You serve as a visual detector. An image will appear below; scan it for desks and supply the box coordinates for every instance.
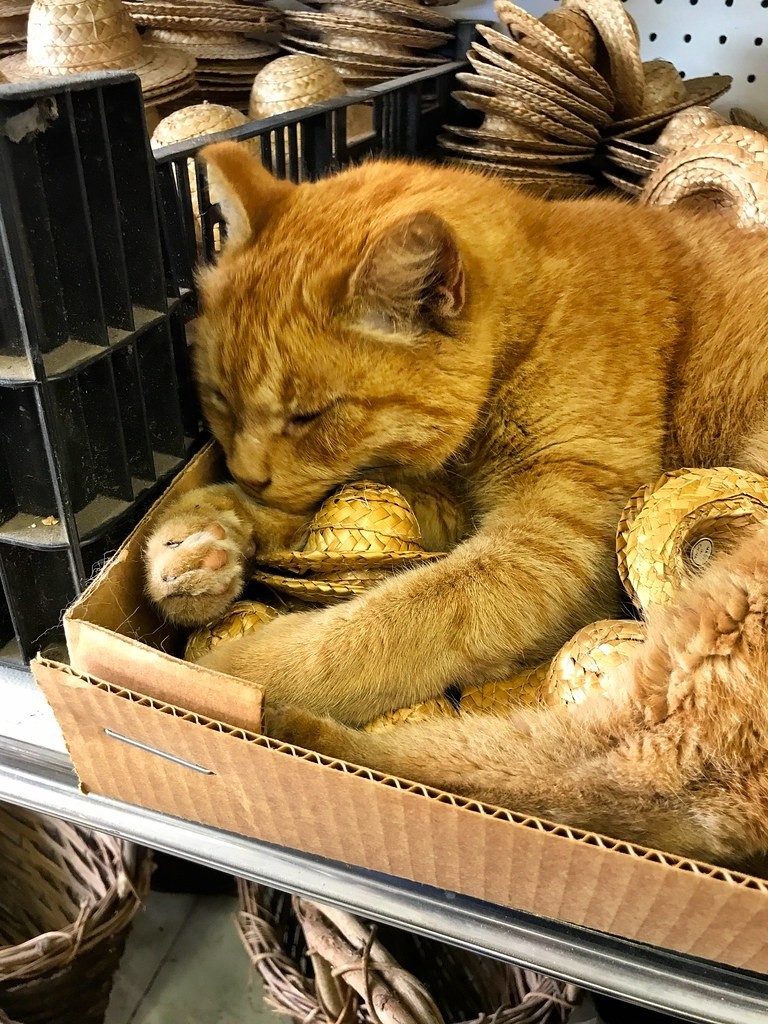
[0,654,768,1024]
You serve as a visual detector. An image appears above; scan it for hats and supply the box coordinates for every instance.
[248,471,449,606]
[457,618,650,719]
[0,0,455,252]
[356,692,461,734]
[434,0,768,230]
[616,465,768,618]
[184,599,286,666]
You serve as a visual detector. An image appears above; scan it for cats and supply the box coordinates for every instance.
[145,142,768,861]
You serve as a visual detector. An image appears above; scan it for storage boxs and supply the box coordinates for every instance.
[28,438,768,975]
[0,61,472,669]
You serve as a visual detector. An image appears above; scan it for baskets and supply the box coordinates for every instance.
[0,800,154,1024]
[229,873,579,1024]
